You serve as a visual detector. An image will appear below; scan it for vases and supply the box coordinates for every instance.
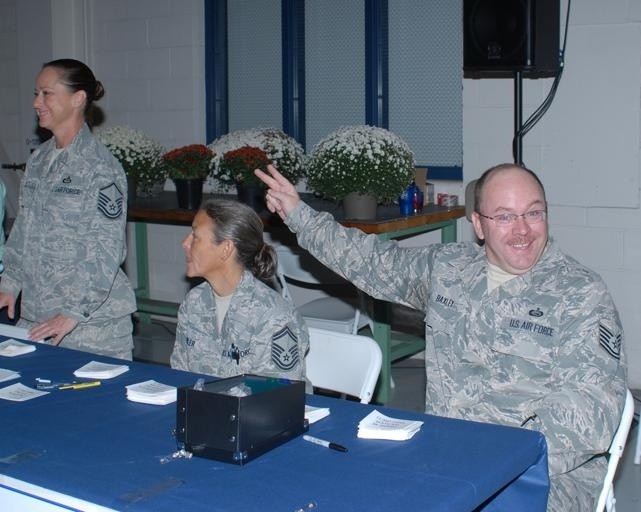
[173,179,205,210]
[126,177,137,205]
[341,190,378,221]
[233,184,265,214]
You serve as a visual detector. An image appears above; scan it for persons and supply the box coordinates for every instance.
[254,164,629,511]
[168,199,313,395]
[1,58,139,361]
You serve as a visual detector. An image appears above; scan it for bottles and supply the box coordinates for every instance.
[399,181,424,219]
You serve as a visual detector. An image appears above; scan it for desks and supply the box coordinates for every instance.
[119,190,463,406]
[0,332,551,512]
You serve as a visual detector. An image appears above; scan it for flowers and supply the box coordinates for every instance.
[160,143,214,179]
[208,130,306,187]
[303,124,416,202]
[91,123,167,184]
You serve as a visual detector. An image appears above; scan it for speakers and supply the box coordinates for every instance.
[463,0,560,79]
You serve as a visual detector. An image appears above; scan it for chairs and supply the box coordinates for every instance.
[591,388,633,512]
[302,326,384,407]
[270,243,395,403]
[0,323,47,344]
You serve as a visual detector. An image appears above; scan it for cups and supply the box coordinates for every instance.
[437,193,458,208]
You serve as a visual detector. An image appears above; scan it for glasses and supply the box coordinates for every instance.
[478,206,550,228]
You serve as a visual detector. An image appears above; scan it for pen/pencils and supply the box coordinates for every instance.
[59,380,101,390]
[303,434,348,452]
[34,383,72,390]
